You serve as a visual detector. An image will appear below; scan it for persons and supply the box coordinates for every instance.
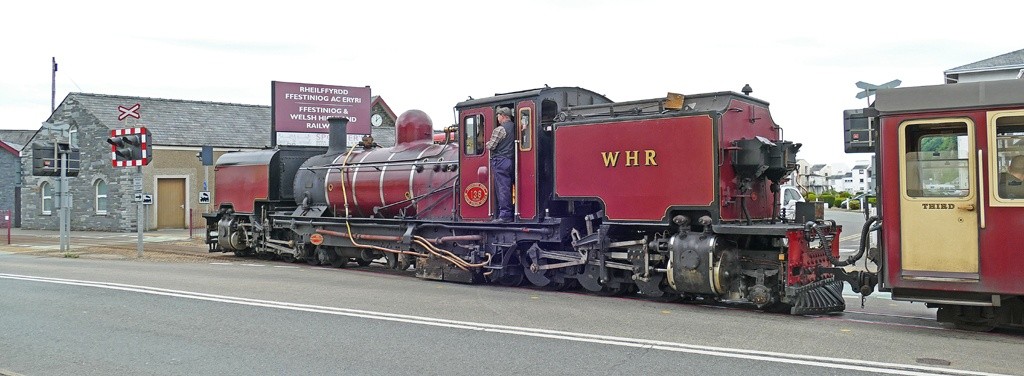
[486,107,515,222]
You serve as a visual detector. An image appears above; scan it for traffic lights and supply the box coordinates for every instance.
[107,127,153,168]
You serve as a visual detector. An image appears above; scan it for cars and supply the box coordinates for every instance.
[841,192,874,210]
[780,184,806,222]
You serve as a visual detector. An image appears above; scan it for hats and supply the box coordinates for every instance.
[496,107,512,116]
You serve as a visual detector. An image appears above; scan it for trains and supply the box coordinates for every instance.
[199,77,1024,340]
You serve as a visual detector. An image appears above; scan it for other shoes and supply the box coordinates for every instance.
[492,218,511,223]
